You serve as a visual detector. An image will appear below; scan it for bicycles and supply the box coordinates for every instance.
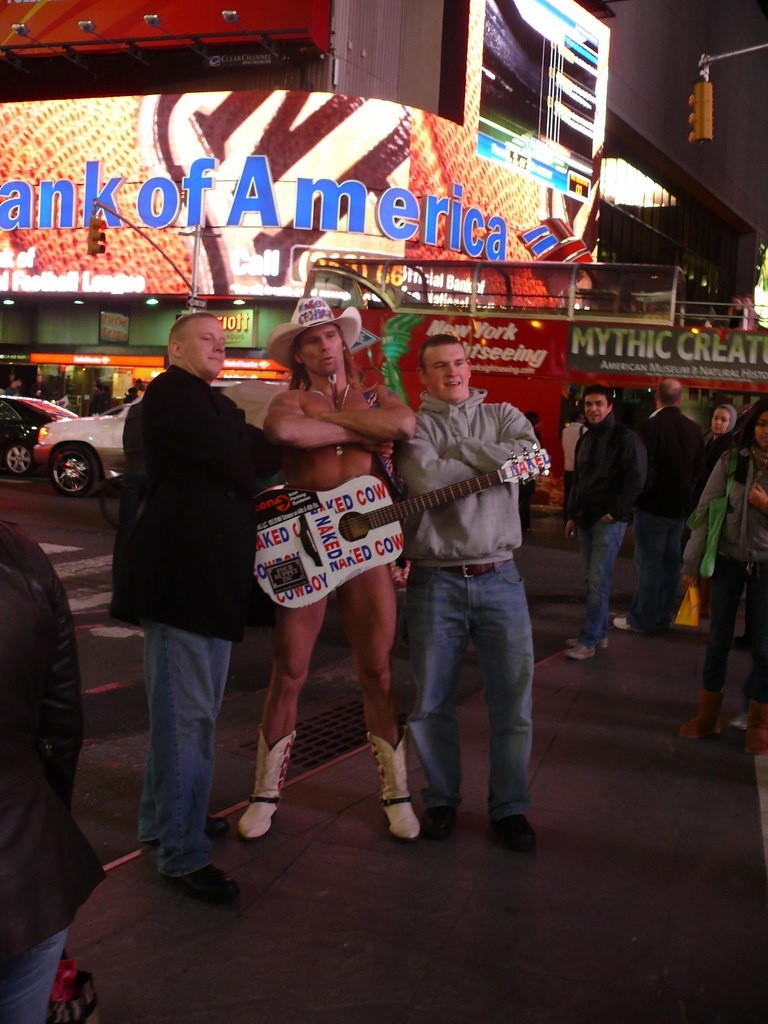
[99,472,127,531]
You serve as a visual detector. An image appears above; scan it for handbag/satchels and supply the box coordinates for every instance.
[673,579,702,628]
[698,448,737,577]
[44,948,98,1024]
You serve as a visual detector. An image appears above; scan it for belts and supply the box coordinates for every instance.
[436,559,507,580]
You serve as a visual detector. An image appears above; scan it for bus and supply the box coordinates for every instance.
[294,250,768,517]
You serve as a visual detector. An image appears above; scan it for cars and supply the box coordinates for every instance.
[0,395,84,479]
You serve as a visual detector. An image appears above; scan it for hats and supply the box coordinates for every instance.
[267,296,362,368]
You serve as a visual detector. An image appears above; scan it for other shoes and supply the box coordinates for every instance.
[566,639,608,660]
[613,618,645,632]
[202,814,231,839]
[491,814,537,850]
[422,805,458,842]
[152,862,241,904]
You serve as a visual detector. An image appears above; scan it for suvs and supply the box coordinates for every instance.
[30,382,292,496]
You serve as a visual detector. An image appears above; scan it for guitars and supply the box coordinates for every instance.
[253,443,550,612]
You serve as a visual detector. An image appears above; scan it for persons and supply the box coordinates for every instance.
[680,399,768,754]
[739,293,757,329]
[388,332,541,854]
[30,374,51,401]
[88,382,111,416]
[705,403,737,480]
[4,377,22,397]
[124,378,146,404]
[613,378,707,635]
[106,308,284,906]
[238,294,423,844]
[519,411,542,532]
[562,405,589,526]
[565,384,649,660]
[727,295,743,330]
[0,522,109,1024]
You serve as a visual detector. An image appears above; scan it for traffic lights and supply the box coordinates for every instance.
[689,80,715,145]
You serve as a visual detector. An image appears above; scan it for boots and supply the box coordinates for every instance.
[238,725,296,841]
[744,697,768,755]
[678,688,724,738]
[367,725,421,842]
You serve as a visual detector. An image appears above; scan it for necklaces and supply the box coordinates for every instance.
[308,384,351,455]
